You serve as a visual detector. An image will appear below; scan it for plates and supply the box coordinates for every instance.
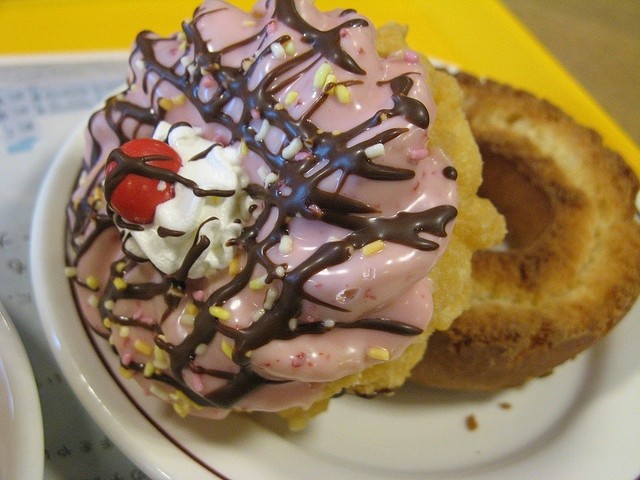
[0,300,46,480]
[30,85,640,480]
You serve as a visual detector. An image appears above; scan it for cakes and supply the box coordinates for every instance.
[65,1,507,431]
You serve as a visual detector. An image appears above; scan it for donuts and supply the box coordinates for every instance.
[407,66,640,391]
